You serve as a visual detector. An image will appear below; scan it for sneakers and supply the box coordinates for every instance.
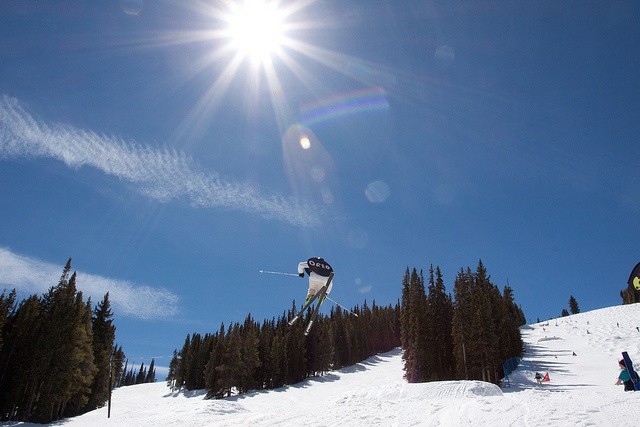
[316,293,325,305]
[305,293,314,305]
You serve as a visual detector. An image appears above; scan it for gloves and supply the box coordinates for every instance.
[299,273,304,278]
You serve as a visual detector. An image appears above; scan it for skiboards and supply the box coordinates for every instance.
[287,272,334,336]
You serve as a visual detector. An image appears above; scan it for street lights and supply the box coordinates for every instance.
[108,350,113,418]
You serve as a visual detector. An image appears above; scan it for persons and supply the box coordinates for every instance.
[632,274,640,303]
[298,257,333,304]
[614,359,640,391]
[534,372,542,385]
